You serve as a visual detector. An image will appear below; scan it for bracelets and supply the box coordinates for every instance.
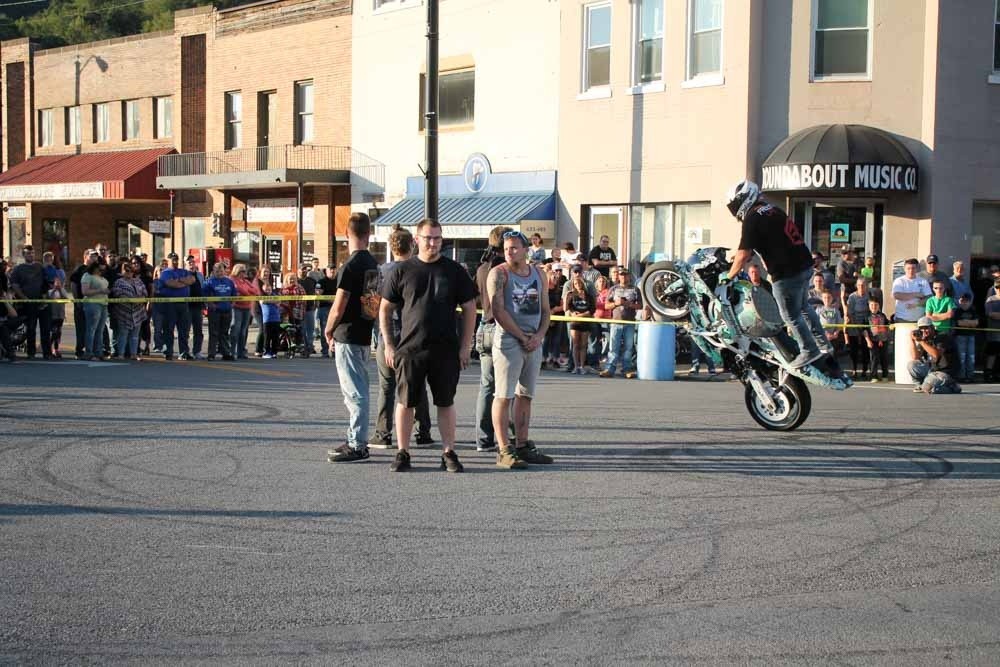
[614,302,616,307]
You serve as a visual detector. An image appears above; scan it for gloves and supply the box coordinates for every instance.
[718,271,733,285]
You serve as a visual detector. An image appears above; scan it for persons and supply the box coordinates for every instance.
[327,212,553,473]
[730,244,1000,383]
[907,317,962,393]
[718,179,833,369]
[460,233,720,379]
[0,240,378,365]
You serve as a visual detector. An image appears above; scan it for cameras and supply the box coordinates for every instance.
[913,330,922,337]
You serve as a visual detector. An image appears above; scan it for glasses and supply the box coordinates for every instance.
[555,269,562,272]
[503,231,527,244]
[417,234,442,241]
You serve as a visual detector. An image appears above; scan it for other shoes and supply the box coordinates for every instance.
[688,358,741,382]
[27,349,335,360]
[541,361,635,378]
[852,371,888,383]
[913,386,925,392]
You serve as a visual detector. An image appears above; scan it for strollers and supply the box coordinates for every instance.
[276,326,310,358]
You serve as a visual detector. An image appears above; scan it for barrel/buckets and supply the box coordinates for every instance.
[635,321,676,380]
[893,323,927,385]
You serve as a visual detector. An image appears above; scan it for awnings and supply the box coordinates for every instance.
[373,193,555,243]
[0,147,175,200]
[761,123,918,198]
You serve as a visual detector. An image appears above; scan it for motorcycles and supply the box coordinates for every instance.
[640,246,811,431]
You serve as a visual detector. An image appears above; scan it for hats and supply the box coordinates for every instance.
[918,317,933,326]
[573,265,582,273]
[552,263,562,270]
[298,263,336,269]
[84,248,98,256]
[927,255,938,264]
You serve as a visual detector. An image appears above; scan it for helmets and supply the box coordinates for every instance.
[727,181,768,221]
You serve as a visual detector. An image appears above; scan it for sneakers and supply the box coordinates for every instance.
[790,349,823,369]
[367,430,392,448]
[819,344,834,353]
[414,434,438,447]
[328,439,370,463]
[477,442,499,452]
[390,449,412,471]
[496,444,528,469]
[440,450,464,473]
[515,440,554,464]
